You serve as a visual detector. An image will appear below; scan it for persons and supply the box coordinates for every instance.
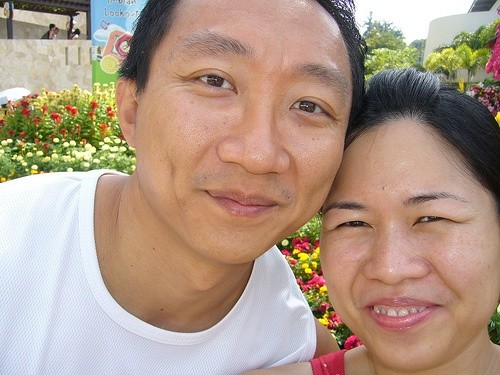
[0,0,370,374]
[41,23,80,40]
[236,67,500,375]
